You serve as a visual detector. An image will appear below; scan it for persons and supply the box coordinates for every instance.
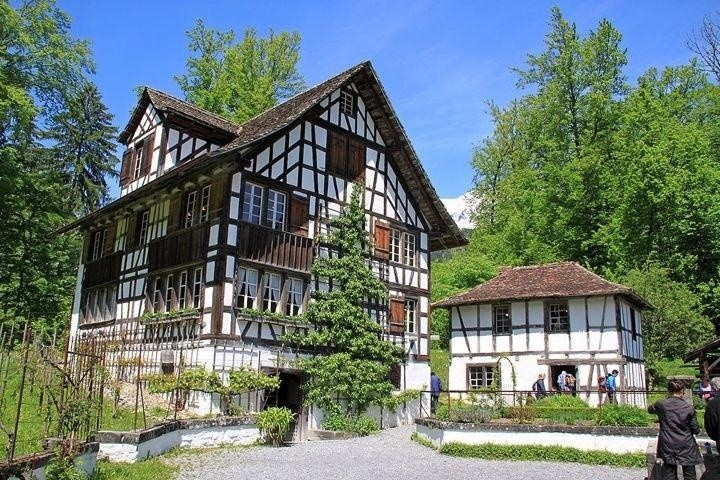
[704,376,720,454]
[431,371,443,414]
[648,379,701,480]
[532,373,547,399]
[557,370,575,395]
[606,370,619,405]
